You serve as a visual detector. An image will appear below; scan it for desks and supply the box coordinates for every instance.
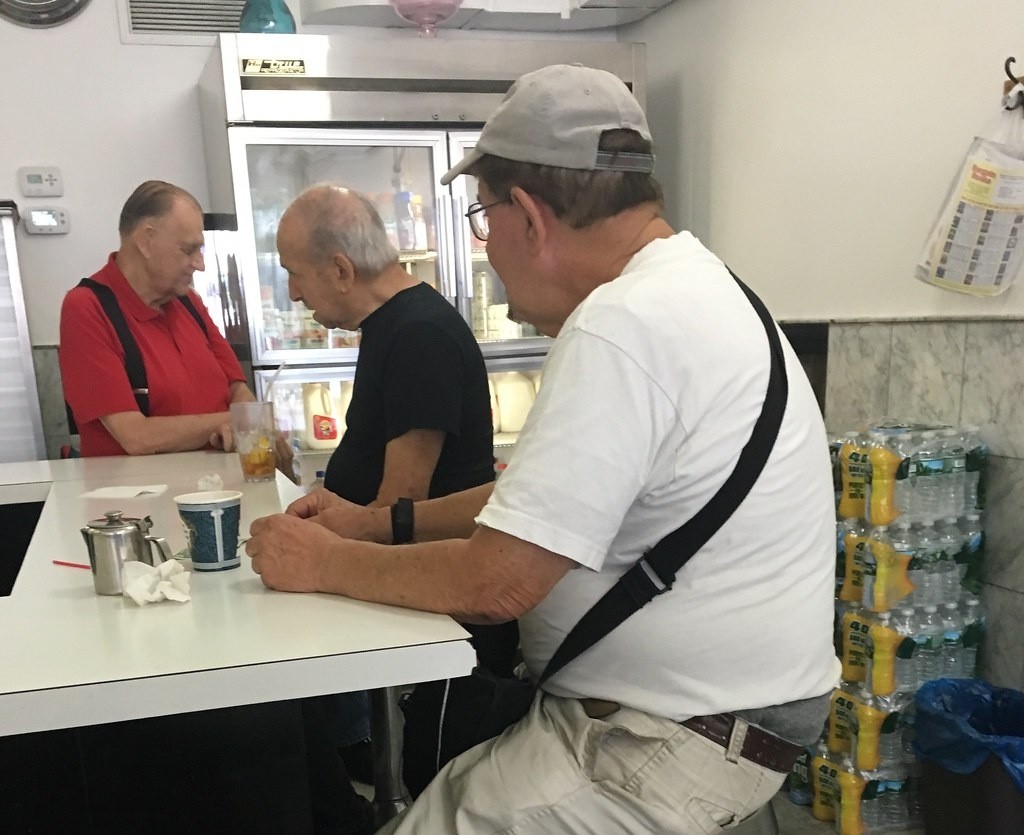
[0,449,479,831]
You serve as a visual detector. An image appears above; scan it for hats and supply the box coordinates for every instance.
[440,62,657,185]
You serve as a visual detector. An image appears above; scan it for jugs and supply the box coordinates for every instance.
[80,511,173,595]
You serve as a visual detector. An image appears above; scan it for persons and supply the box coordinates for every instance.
[245,63,842,835]
[59,180,294,466]
[276,184,496,508]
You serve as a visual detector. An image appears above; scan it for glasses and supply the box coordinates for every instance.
[465,198,509,241]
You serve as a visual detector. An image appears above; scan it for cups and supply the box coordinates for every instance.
[230,402,276,482]
[173,491,243,572]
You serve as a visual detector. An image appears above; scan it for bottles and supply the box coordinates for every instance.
[239,0,297,34]
[487,370,542,433]
[783,407,987,834]
[250,183,440,350]
[308,471,325,491]
[301,380,356,450]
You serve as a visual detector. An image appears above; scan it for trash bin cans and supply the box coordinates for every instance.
[916,679,1024,835]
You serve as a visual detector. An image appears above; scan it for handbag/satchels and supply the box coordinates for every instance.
[406,666,536,802]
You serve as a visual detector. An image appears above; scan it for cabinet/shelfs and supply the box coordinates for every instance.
[229,118,560,476]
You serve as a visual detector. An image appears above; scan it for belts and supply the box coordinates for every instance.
[580,698,803,772]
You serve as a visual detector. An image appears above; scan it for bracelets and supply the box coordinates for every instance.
[390,498,414,543]
